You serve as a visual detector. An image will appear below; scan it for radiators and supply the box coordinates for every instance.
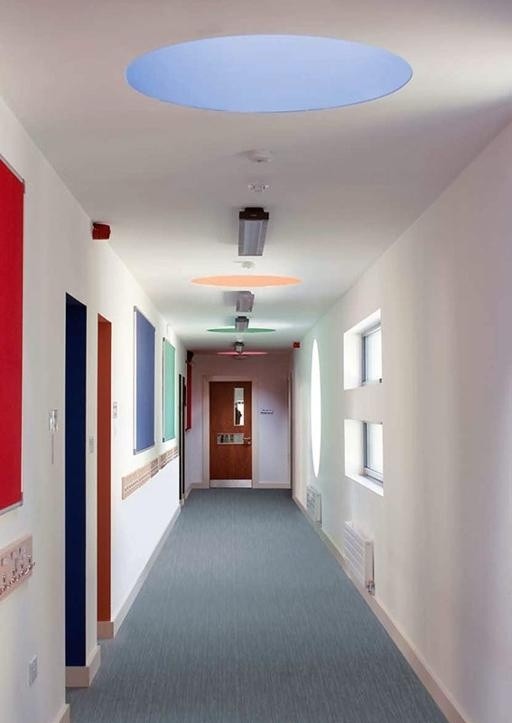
[304,485,322,525]
[340,519,375,598]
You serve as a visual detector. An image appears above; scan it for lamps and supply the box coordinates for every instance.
[233,316,250,333]
[235,202,270,258]
[233,341,245,354]
[233,291,255,312]
